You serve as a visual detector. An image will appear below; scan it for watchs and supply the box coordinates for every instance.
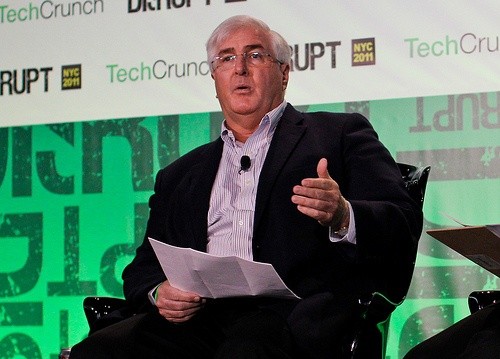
[331,200,351,237]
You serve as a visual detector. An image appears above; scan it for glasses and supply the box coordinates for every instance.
[209,52,284,69]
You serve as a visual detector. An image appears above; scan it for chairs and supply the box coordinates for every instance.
[56,163,431,359]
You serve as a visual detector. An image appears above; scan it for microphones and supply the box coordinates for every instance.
[238,155,251,175]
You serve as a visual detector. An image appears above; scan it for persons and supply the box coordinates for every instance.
[403,297,500,359]
[66,13,424,359]
